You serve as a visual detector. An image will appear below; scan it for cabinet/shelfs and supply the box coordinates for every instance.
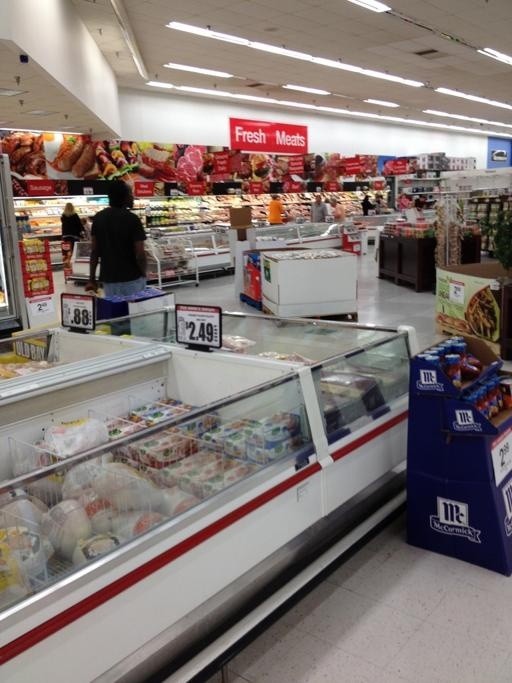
[0,304,423,683]
[405,334,512,577]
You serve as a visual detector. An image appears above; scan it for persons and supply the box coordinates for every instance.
[311,191,332,222]
[373,193,387,215]
[361,195,376,216]
[268,194,292,225]
[86,179,146,296]
[330,198,345,222]
[413,196,426,209]
[394,191,411,213]
[60,202,97,261]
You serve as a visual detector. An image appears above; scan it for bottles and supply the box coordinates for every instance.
[413,334,512,422]
[144,199,184,226]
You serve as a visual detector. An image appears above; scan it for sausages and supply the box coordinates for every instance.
[72,145,94,177]
[58,140,82,170]
